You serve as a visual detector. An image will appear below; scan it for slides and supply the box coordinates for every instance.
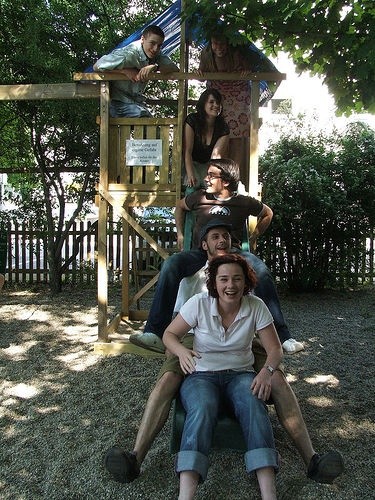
[168,187,250,453]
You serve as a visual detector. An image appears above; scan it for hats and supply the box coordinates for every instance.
[199,220,233,240]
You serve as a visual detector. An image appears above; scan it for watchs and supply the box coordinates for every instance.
[262,365,275,376]
[154,62,161,72]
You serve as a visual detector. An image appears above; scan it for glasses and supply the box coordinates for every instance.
[206,173,225,179]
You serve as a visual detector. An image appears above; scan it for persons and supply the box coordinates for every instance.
[197,28,259,181]
[92,24,180,182]
[102,218,345,485]
[162,252,285,500]
[181,88,232,193]
[128,158,306,355]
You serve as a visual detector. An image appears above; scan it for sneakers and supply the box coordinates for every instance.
[129,332,166,354]
[282,338,305,355]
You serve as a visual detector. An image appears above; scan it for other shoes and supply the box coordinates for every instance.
[104,448,140,483]
[309,450,344,483]
